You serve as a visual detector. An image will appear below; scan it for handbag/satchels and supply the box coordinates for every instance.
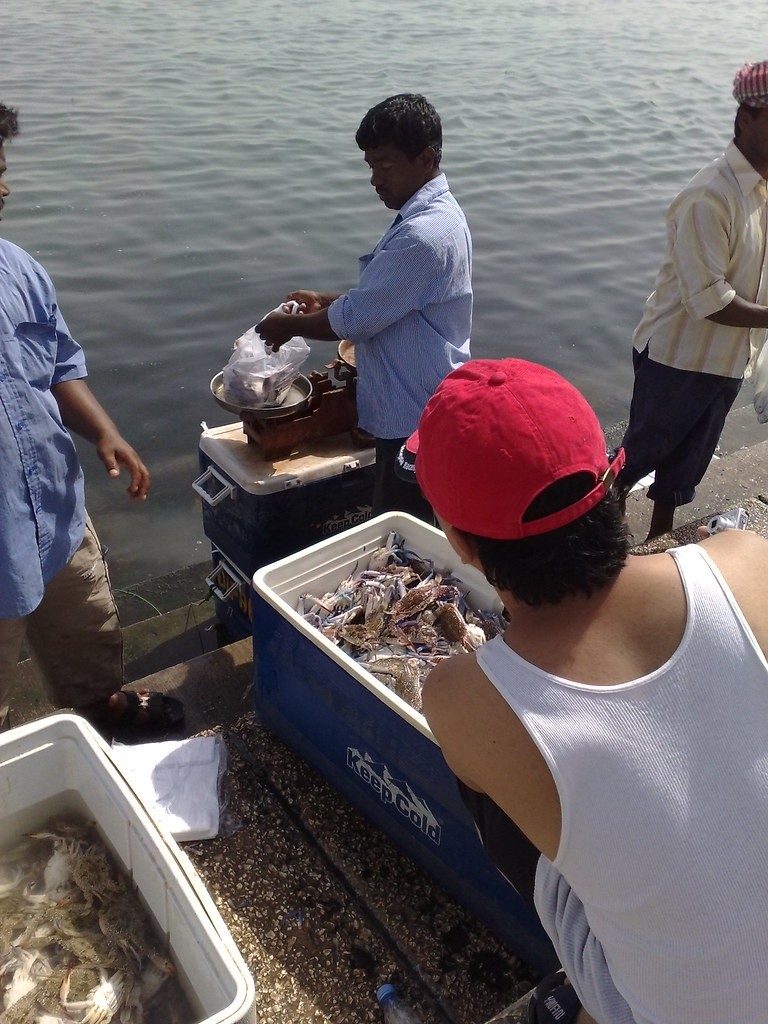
[224,299,308,408]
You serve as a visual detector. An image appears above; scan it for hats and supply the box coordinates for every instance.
[393,359,626,540]
[732,59,768,107]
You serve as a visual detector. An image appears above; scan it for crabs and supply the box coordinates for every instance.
[0,817,174,1024]
[296,532,509,713]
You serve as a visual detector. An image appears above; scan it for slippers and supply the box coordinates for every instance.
[528,972,584,1024]
[96,691,185,730]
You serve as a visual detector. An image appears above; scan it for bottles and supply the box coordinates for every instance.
[376,983,424,1024]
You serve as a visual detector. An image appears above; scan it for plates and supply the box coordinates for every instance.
[210,368,313,417]
[338,338,356,371]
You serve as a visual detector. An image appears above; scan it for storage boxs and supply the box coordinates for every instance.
[191,420,376,579]
[0,713,256,1024]
[251,512,561,976]
[206,541,253,640]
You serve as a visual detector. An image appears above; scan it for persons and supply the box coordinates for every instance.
[609,59,768,543]
[254,94,475,530]
[0,101,187,737]
[405,356,768,1024]
